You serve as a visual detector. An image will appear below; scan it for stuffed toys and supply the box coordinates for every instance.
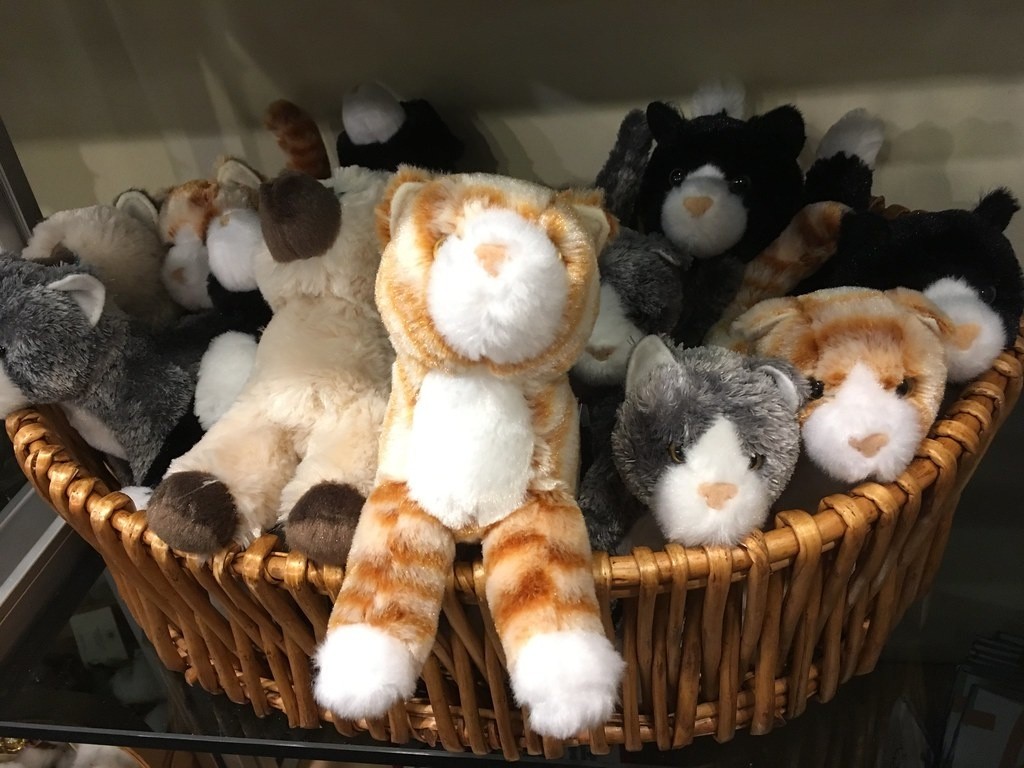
[0,76,1024,738]
[311,163,632,738]
[145,163,389,566]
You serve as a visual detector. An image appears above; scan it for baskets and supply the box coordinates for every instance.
[6,315,1024,761]
